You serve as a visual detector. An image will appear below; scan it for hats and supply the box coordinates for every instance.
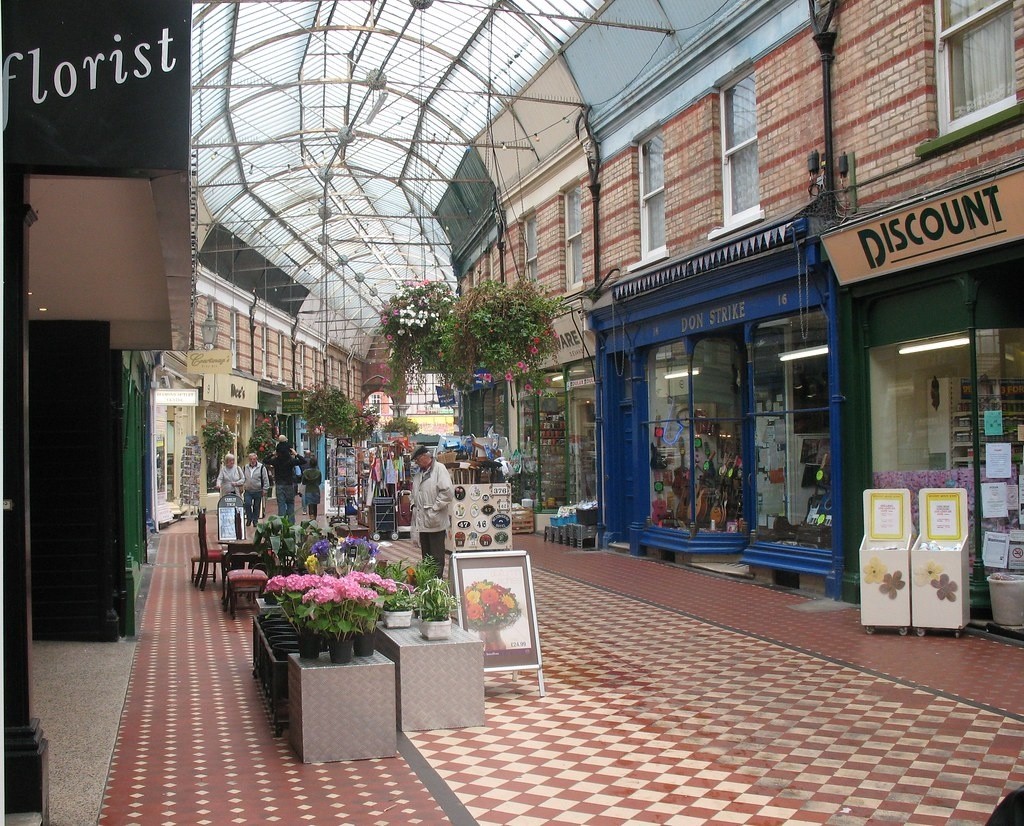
[410,446,428,461]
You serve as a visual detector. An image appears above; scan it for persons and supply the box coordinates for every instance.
[410,446,455,578]
[217,453,269,527]
[263,435,322,525]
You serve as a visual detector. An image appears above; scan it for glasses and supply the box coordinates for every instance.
[414,455,422,462]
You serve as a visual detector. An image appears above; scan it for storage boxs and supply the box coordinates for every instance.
[549,507,598,526]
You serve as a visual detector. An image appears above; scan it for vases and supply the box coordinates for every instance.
[329,639,353,664]
[298,639,320,658]
[255,613,303,661]
[312,633,327,652]
[352,632,374,657]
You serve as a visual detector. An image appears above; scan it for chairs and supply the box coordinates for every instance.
[195,507,230,591]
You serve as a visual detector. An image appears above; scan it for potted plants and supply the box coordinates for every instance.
[380,581,416,629]
[418,588,453,640]
[420,578,453,616]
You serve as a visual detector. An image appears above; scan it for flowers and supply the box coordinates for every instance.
[265,572,398,640]
[303,535,381,577]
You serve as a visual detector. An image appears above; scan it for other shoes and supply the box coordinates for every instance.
[303,512,307,515]
[253,523,257,527]
[246,520,252,526]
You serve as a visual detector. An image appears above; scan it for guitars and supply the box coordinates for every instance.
[803,454,832,526]
[651,436,732,529]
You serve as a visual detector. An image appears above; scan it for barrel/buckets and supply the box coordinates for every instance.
[987,575,1024,625]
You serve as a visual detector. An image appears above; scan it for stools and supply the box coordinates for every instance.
[190,556,216,584]
[222,566,268,620]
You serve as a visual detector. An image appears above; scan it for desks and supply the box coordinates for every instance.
[217,540,266,598]
